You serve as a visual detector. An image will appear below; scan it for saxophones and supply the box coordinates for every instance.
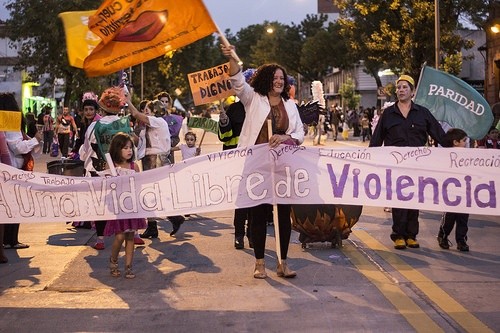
[24,125,44,157]
[110,71,131,109]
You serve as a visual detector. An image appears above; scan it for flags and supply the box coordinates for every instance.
[60,10,102,68]
[82,0,220,77]
[414,65,494,140]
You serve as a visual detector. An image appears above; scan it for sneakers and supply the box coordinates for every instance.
[406,238,419,247]
[457,241,469,251]
[394,239,406,248]
[439,239,449,249]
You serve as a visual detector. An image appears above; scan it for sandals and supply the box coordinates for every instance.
[277,259,297,276]
[110,256,120,276]
[254,259,266,278]
[3,240,29,248]
[125,265,135,278]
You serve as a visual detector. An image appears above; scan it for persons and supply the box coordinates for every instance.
[360,74,448,250]
[25,106,85,157]
[471,102,500,149]
[300,100,386,147]
[0,131,12,263]
[219,68,255,249]
[103,131,147,279]
[437,128,472,252]
[156,93,183,164]
[126,93,185,239]
[217,43,304,279]
[133,100,150,136]
[171,132,201,217]
[91,87,145,250]
[67,93,102,229]
[267,75,308,226]
[55,106,79,158]
[0,92,44,248]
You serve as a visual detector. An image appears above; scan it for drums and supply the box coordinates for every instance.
[45,158,85,178]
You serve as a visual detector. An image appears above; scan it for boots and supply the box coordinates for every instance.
[167,215,185,236]
[139,221,158,238]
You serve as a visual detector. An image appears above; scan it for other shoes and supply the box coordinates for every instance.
[0,255,8,263]
[95,239,104,249]
[250,240,254,248]
[235,239,244,248]
[134,235,145,244]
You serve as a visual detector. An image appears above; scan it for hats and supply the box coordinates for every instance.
[397,75,414,86]
